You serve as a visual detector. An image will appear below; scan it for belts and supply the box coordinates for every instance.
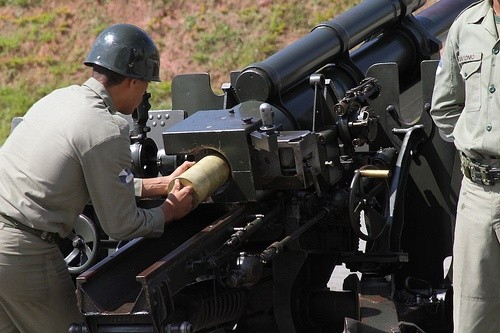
[459,150,500,186]
[0,212,59,244]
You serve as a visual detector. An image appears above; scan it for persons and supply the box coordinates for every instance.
[428,0,499,333]
[0,23,196,333]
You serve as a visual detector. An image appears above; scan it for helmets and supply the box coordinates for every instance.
[83,23,162,82]
